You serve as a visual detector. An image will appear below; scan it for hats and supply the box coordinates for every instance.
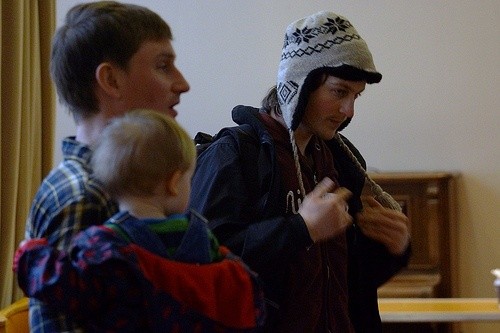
[276,10,403,211]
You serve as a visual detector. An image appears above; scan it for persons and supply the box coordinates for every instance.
[12,0,268,333]
[190,11,413,333]
